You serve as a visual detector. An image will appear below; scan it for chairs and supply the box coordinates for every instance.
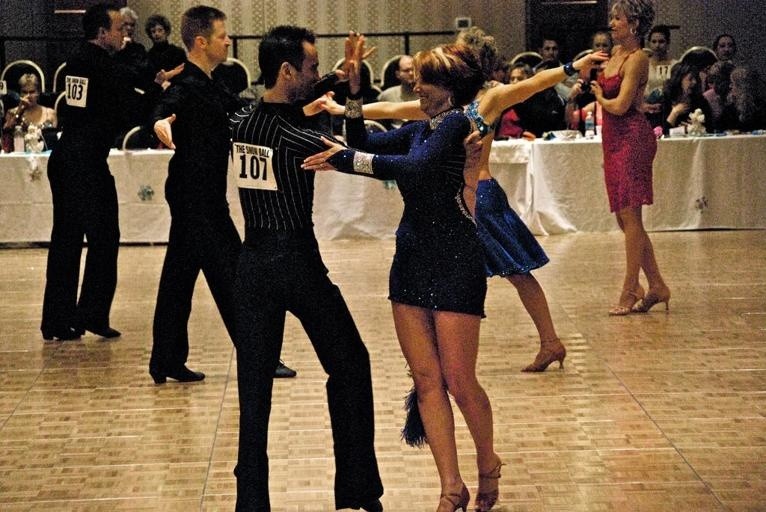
[679,46,718,70]
[1,60,46,95]
[0,134,766,246]
[379,54,402,92]
[511,52,543,67]
[55,91,66,125]
[573,49,592,61]
[364,119,388,133]
[122,125,158,149]
[53,63,67,92]
[210,57,251,94]
[331,58,381,106]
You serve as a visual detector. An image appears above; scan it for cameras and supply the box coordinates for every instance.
[581,80,592,92]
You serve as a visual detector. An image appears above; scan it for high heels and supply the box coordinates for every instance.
[440,483,470,512]
[336,484,383,512]
[520,337,565,372]
[631,286,671,312]
[474,456,502,512]
[42,326,82,341]
[608,285,645,316]
[149,362,205,384]
[275,362,297,378]
[75,318,121,337]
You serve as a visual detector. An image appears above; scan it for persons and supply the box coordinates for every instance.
[119,5,144,59]
[491,23,764,143]
[3,72,57,132]
[37,2,165,343]
[297,43,506,512]
[144,5,296,386]
[375,54,420,102]
[318,24,611,373]
[226,23,388,511]
[589,1,674,318]
[142,13,184,70]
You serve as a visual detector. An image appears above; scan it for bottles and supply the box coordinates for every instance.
[585,112,595,141]
[14,126,24,152]
